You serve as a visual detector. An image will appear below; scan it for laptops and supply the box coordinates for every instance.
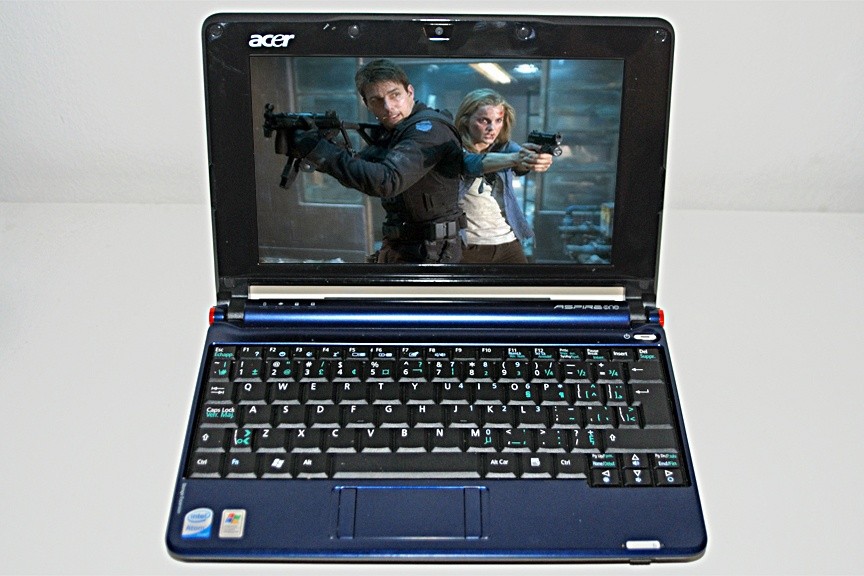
[165,11,708,567]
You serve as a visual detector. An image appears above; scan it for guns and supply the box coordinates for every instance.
[263,102,385,191]
[526,128,565,160]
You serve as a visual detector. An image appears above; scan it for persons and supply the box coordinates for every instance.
[292,58,465,263]
[455,87,553,263]
